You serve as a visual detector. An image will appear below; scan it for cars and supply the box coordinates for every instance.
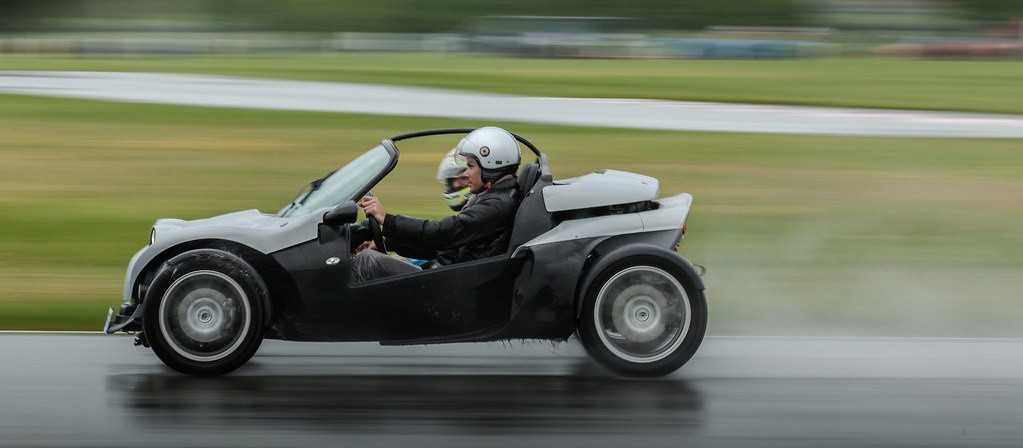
[99,124,711,380]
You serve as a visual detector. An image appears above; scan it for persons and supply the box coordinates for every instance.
[354,146,478,271]
[349,125,525,283]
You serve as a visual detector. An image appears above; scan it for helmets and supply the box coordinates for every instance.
[458,126,522,184]
[438,147,474,211]
